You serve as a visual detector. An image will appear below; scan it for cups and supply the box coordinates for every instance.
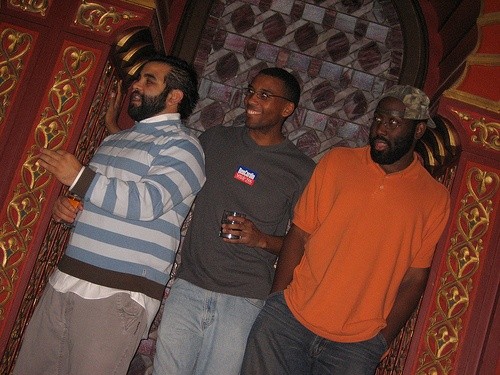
[67,190,81,207]
[221,209,246,239]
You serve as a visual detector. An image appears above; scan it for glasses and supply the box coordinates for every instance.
[369,117,407,129]
[243,88,292,102]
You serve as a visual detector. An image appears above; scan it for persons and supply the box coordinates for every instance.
[150,67,316,374]
[11,56,207,375]
[240,83,450,375]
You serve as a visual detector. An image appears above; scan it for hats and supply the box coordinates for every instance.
[375,83,436,128]
